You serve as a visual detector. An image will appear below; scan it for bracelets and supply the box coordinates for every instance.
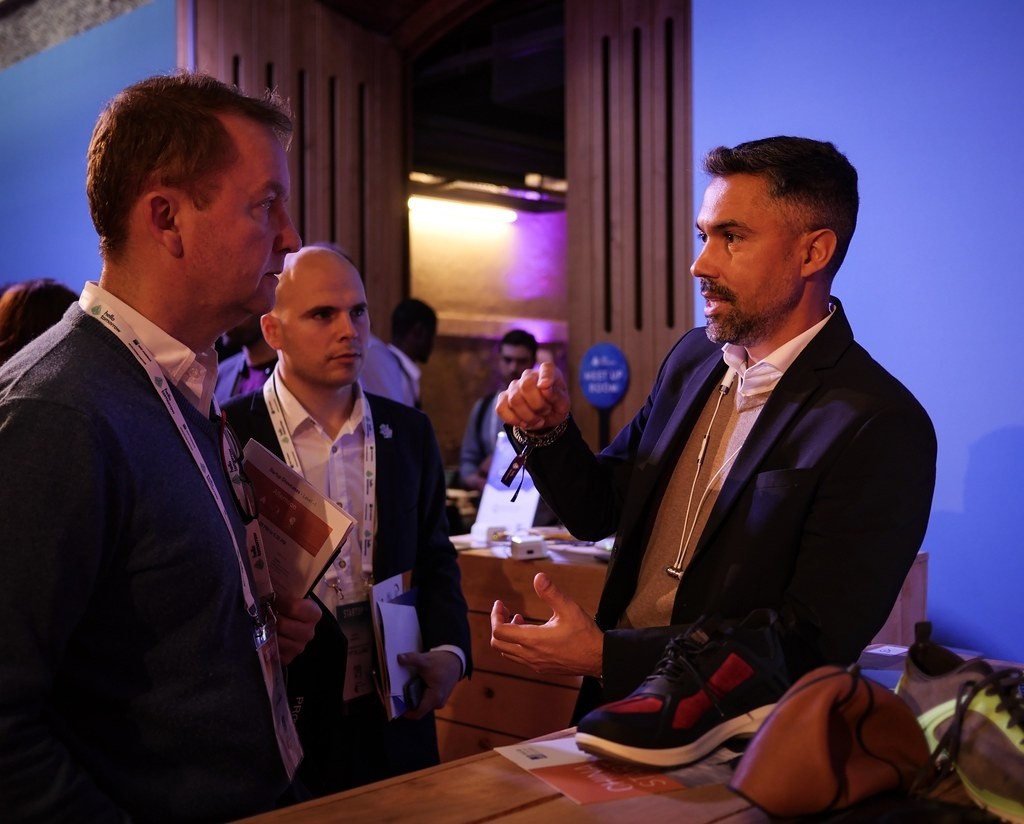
[501,413,573,502]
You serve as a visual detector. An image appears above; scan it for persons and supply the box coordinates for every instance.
[0,279,554,530]
[0,69,304,824]
[221,243,473,798]
[492,136,937,728]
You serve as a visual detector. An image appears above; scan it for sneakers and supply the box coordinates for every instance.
[893,621,1024,824]
[574,613,792,767]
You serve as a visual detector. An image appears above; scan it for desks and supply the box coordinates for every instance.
[226,641,1024,824]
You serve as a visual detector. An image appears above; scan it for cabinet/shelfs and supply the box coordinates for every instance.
[435,526,929,762]
